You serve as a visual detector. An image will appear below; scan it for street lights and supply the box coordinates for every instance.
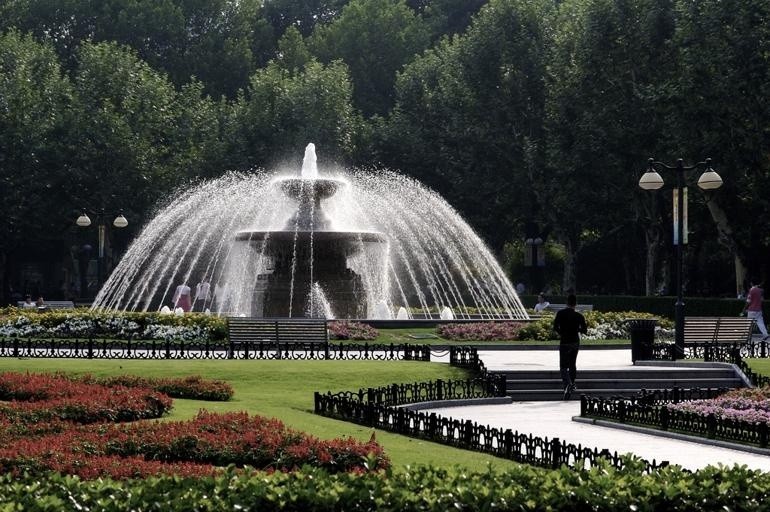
[639,157,727,360]
[525,237,545,297]
[76,206,129,294]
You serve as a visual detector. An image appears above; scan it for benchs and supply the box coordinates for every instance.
[226,316,328,358]
[683,316,755,364]
[546,304,594,317]
[18,300,74,311]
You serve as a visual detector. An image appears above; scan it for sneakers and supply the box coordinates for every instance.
[563,383,577,401]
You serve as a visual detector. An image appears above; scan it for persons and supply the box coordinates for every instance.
[743,279,769,341]
[553,293,587,400]
[533,292,550,312]
[516,280,525,296]
[172,274,229,312]
[22,294,44,309]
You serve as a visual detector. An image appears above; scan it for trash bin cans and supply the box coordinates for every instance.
[626,318,659,365]
